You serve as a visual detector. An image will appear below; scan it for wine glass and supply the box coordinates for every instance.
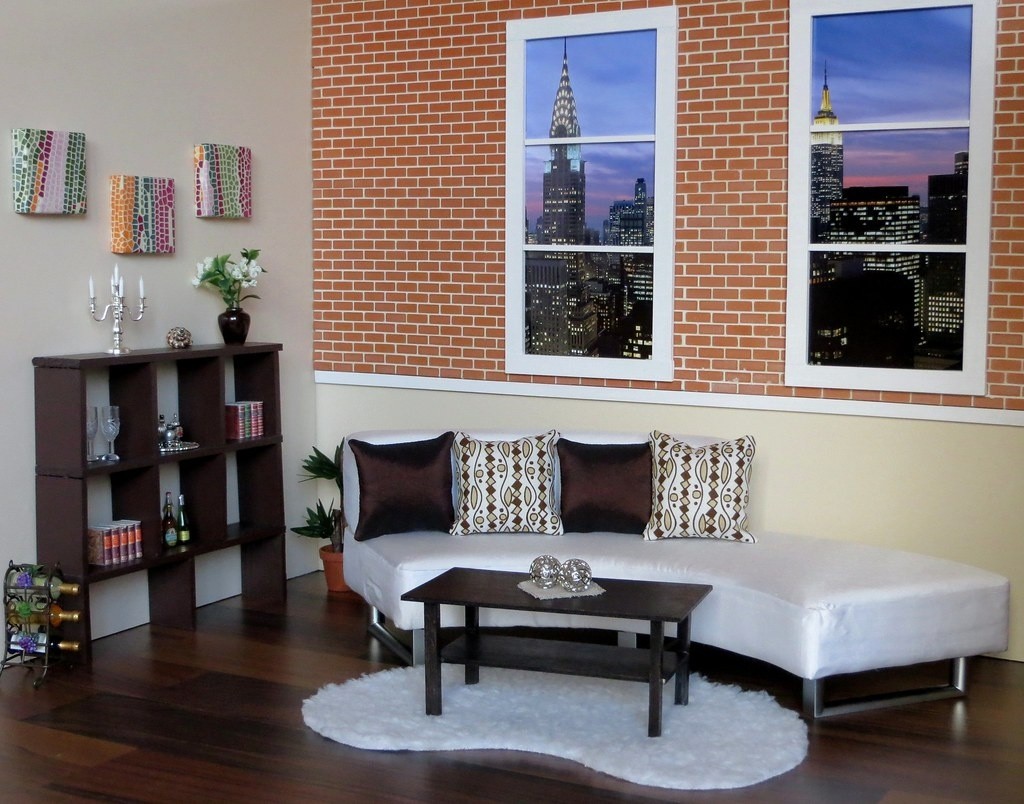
[99,406,122,461]
[84,405,100,461]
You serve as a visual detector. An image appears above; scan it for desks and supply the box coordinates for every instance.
[400,567,713,740]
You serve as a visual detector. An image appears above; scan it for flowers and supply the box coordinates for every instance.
[192,248,268,308]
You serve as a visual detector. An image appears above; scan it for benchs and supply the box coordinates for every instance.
[342,431,1010,721]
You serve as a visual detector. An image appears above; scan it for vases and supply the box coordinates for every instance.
[218,307,251,345]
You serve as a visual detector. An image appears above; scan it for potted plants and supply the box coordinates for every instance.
[290,435,353,592]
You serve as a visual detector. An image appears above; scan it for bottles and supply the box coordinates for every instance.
[158,414,183,442]
[176,493,191,546]
[7,571,80,599]
[7,600,81,626]
[8,631,81,655]
[162,491,179,548]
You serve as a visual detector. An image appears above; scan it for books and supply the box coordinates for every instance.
[88,520,142,566]
[225,401,263,438]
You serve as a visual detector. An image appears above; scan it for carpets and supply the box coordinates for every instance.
[301,660,809,790]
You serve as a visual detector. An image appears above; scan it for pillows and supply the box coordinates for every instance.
[348,431,761,546]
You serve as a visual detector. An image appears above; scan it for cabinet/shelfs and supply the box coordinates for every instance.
[32,340,289,681]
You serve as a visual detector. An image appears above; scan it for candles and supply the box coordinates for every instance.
[89,263,144,297]
